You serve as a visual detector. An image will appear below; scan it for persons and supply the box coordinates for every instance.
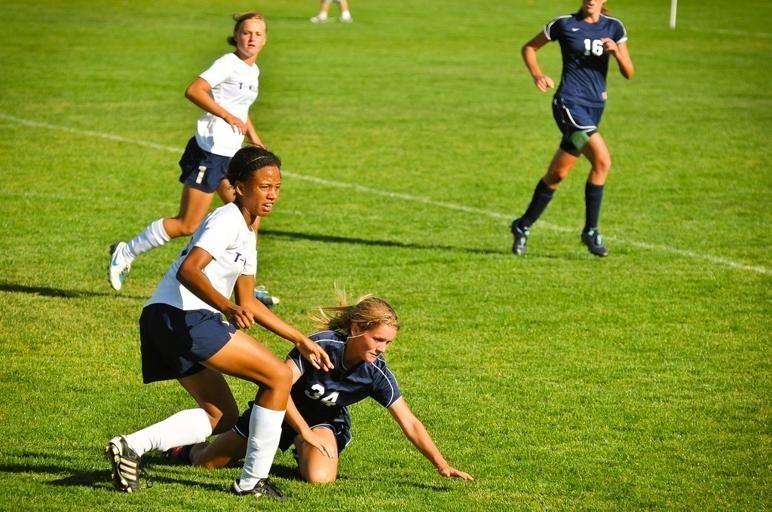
[105,11,281,308]
[101,143,332,502]
[156,282,478,487]
[507,0,635,257]
[307,0,358,24]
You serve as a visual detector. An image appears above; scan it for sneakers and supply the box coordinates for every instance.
[159,442,183,464]
[108,239,134,294]
[105,433,144,497]
[578,226,609,259]
[508,219,530,257]
[228,474,285,499]
[252,284,281,307]
